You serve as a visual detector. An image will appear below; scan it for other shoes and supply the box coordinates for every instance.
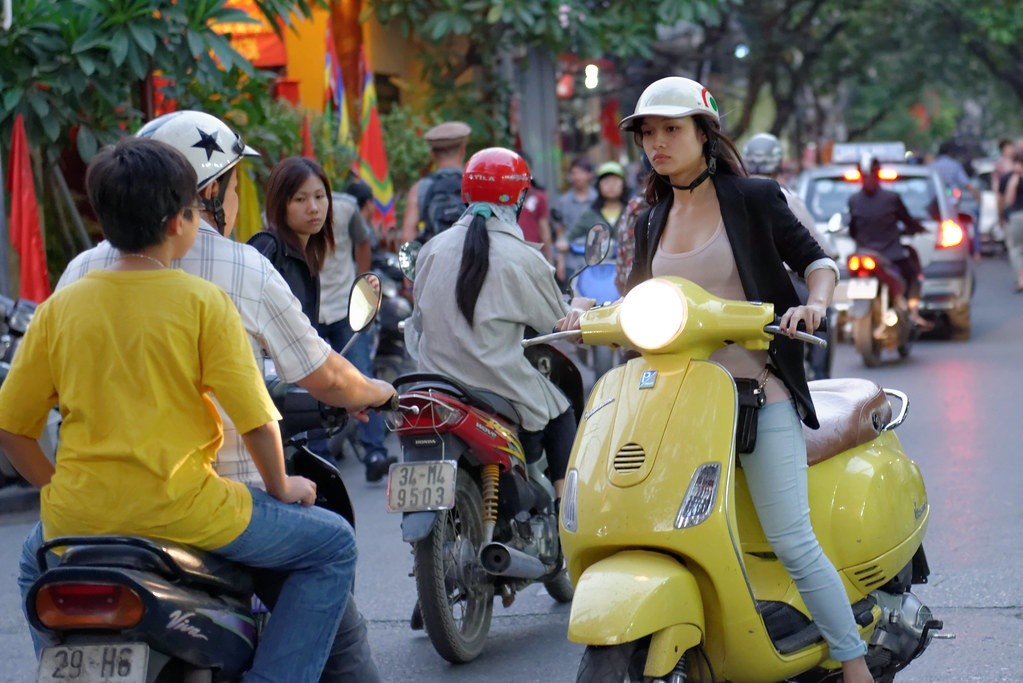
[411,603,424,631]
[908,311,935,329]
[364,452,398,482]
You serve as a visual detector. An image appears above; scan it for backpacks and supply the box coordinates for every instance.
[415,172,468,244]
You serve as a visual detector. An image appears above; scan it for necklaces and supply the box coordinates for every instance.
[120,254,164,267]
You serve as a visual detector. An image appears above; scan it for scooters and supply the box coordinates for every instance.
[0,225,626,683]
[846,227,932,368]
[522,276,956,683]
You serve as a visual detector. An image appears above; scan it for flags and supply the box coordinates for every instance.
[352,47,395,232]
[6,114,51,302]
[323,34,348,147]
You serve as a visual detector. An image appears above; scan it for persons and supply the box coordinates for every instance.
[400,147,595,513]
[743,134,840,381]
[518,152,630,295]
[347,184,379,270]
[558,77,874,682]
[992,139,1023,292]
[245,157,335,335]
[1,139,356,683]
[931,141,982,261]
[400,121,472,302]
[849,154,934,329]
[257,191,385,466]
[54,111,398,682]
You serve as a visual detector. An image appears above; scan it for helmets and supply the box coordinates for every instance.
[616,76,721,132]
[596,161,624,179]
[458,148,530,206]
[132,110,261,192]
[858,154,882,176]
[744,133,781,173]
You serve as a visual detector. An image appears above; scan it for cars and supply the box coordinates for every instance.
[801,140,998,340]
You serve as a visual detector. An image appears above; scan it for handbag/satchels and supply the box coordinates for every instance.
[733,376,766,453]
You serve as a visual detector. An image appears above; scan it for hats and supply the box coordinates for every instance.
[426,122,472,147]
